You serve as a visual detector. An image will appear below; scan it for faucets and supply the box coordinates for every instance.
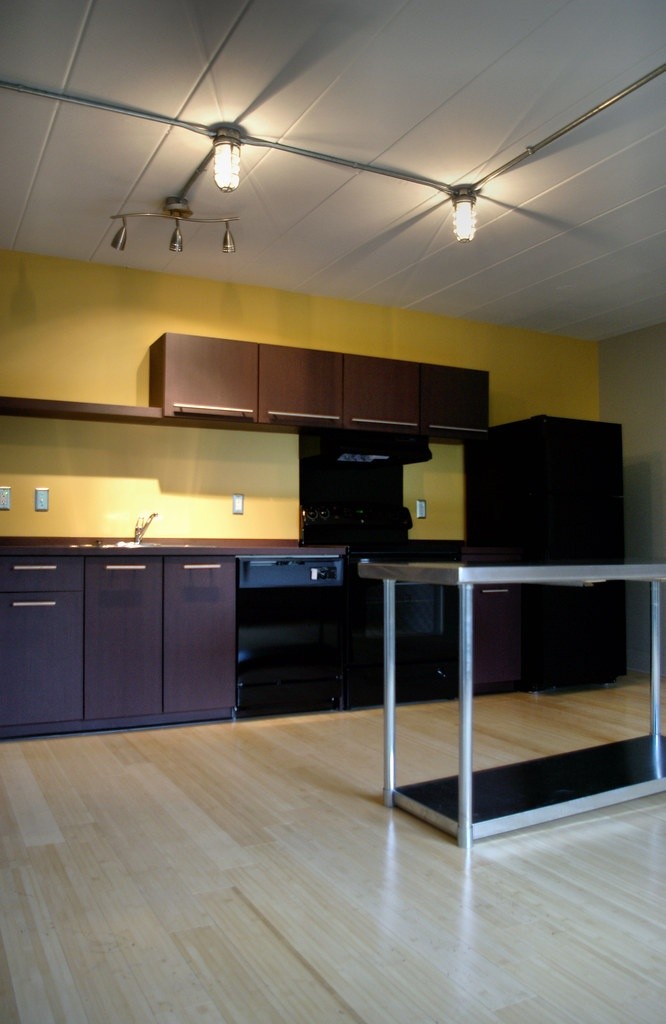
[136,512,158,543]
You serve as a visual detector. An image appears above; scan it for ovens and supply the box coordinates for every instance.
[346,557,460,708]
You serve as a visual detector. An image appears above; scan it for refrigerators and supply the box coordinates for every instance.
[464,415,629,694]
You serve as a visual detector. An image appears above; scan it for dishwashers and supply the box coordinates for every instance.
[233,554,343,718]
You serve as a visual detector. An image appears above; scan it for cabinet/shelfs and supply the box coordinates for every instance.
[463,415,628,693]
[148,332,490,442]
[0,555,236,740]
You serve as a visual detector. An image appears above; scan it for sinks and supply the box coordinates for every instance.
[68,542,212,549]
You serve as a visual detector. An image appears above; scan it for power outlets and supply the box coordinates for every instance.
[0,487,12,511]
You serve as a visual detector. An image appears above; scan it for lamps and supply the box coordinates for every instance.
[212,126,244,194]
[169,218,182,253]
[223,221,236,254]
[451,188,477,243]
[110,217,128,251]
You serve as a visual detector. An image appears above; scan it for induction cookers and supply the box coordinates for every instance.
[302,504,457,558]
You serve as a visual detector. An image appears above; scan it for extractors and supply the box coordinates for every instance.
[299,427,433,466]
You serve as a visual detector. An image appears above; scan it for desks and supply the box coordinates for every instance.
[355,561,665,851]
